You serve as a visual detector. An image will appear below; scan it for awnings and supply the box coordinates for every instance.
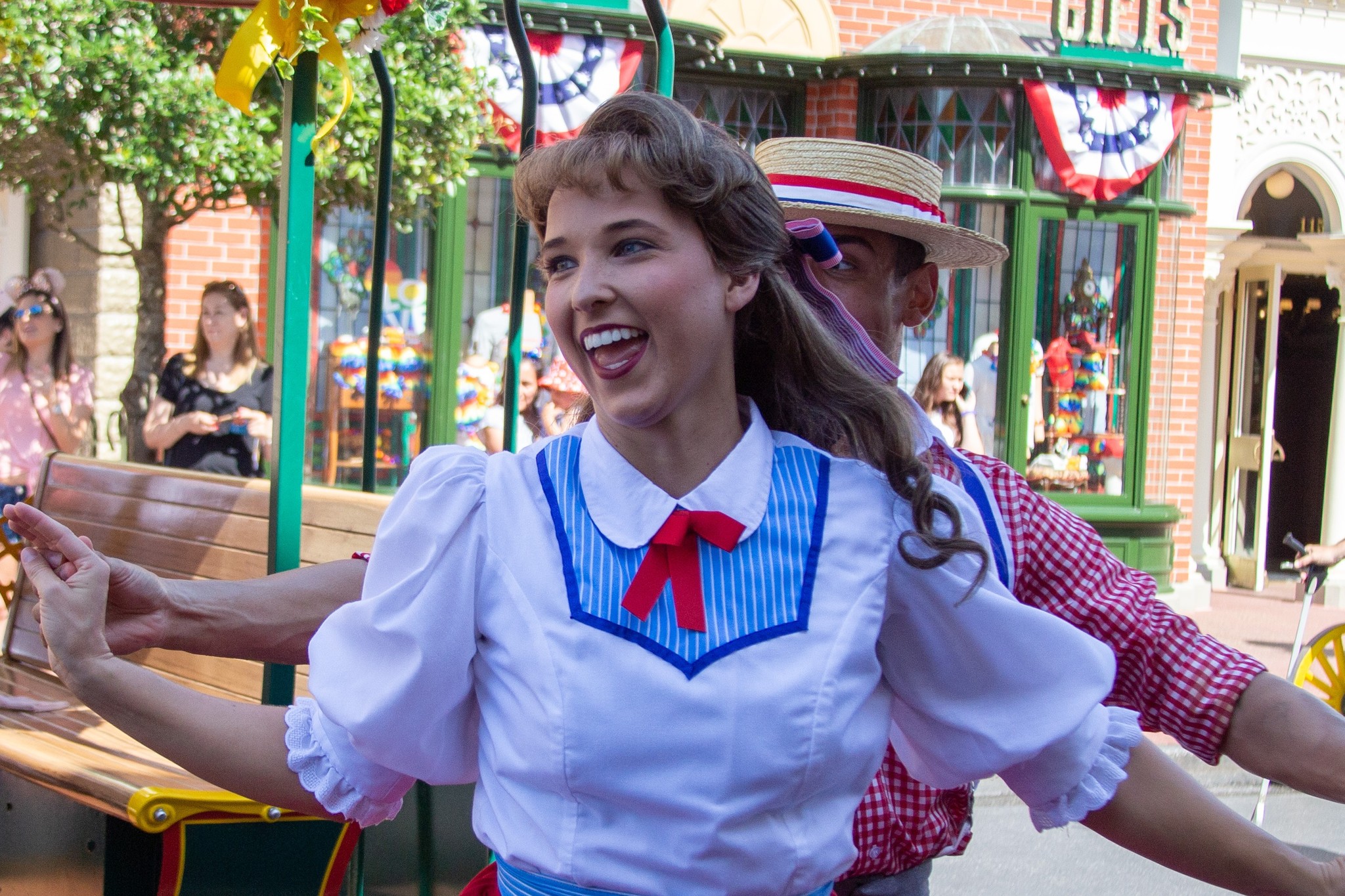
[479,2,1251,105]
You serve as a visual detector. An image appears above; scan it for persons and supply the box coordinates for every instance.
[0,291,94,542]
[0,307,20,354]
[914,351,984,456]
[35,138,1345,896]
[1295,538,1345,584]
[3,93,1345,896]
[142,281,275,478]
[537,354,587,440]
[482,351,545,454]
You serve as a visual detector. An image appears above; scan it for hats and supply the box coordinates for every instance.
[756,136,1010,270]
[538,358,587,396]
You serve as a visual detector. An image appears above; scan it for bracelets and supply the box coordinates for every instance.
[170,417,186,440]
[39,401,63,421]
[959,411,977,418]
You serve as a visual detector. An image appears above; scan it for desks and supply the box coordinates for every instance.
[322,348,427,487]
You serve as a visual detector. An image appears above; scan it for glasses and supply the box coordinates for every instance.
[13,303,56,318]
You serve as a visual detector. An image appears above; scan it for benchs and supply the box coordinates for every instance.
[0,449,399,896]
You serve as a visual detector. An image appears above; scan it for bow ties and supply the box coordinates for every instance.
[621,510,748,634]
